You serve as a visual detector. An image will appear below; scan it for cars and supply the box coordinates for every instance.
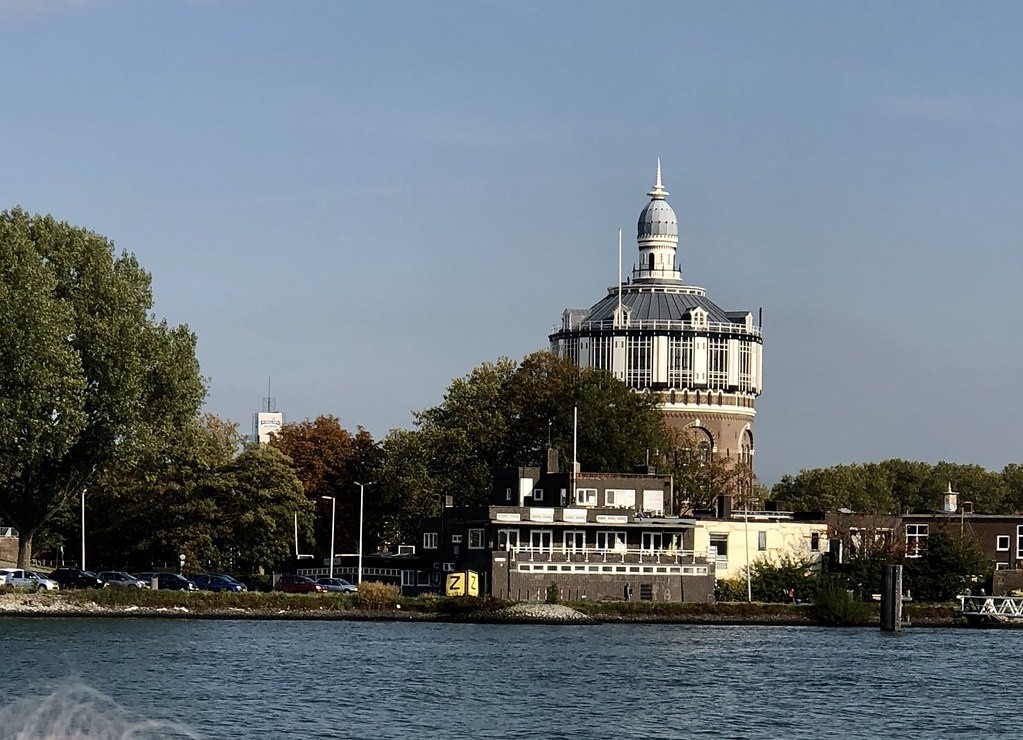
[137,572,199,591]
[194,574,248,592]
[315,578,358,595]
[97,571,150,591]
[276,576,328,594]
[0,568,24,587]
[5,570,60,592]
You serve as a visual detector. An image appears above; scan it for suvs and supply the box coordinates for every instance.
[48,567,110,589]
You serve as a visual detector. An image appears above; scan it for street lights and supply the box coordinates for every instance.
[322,496,336,583]
[353,481,363,584]
[82,489,89,570]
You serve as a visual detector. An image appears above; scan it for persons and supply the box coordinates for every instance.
[978,588,988,613]
[959,588,974,612]
[624,583,632,602]
